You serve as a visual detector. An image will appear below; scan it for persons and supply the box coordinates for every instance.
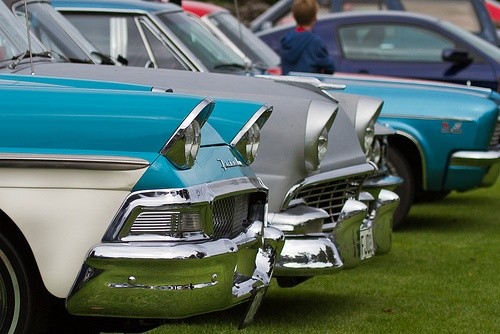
[278,0,336,76]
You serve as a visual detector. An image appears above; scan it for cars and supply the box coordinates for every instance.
[1,0,498,334]
